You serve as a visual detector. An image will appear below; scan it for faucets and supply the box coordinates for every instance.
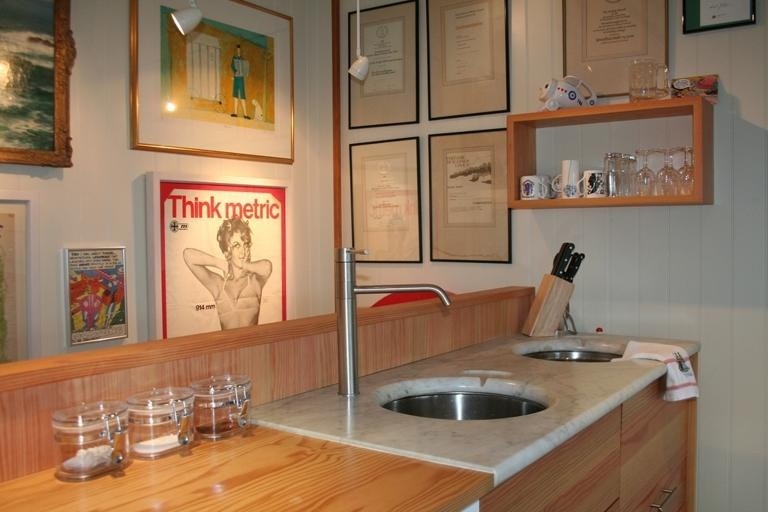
[334,245,452,398]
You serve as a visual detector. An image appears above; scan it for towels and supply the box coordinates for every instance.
[611,340,700,401]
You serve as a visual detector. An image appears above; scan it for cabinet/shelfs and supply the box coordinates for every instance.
[2,284,696,512]
[505,95,713,209]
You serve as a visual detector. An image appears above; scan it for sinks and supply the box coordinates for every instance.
[371,374,556,421]
[509,338,628,364]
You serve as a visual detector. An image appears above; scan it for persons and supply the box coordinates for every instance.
[230,44,251,119]
[184,218,272,330]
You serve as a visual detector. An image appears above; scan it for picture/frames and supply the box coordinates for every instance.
[563,1,669,99]
[64,245,130,346]
[143,171,287,342]
[129,0,296,166]
[348,136,422,265]
[0,198,27,363]
[0,0,77,168]
[427,129,515,265]
[680,0,760,36]
[347,2,419,130]
[426,0,512,120]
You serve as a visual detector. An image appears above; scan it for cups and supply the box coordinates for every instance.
[519,176,547,201]
[552,159,582,199]
[654,145,679,196]
[575,169,607,198]
[604,153,623,197]
[685,148,694,195]
[635,148,655,196]
[628,58,669,101]
[620,154,636,195]
[537,174,556,198]
[680,145,688,192]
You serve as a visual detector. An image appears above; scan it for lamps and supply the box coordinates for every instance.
[172,0,206,36]
[347,0,370,82]
